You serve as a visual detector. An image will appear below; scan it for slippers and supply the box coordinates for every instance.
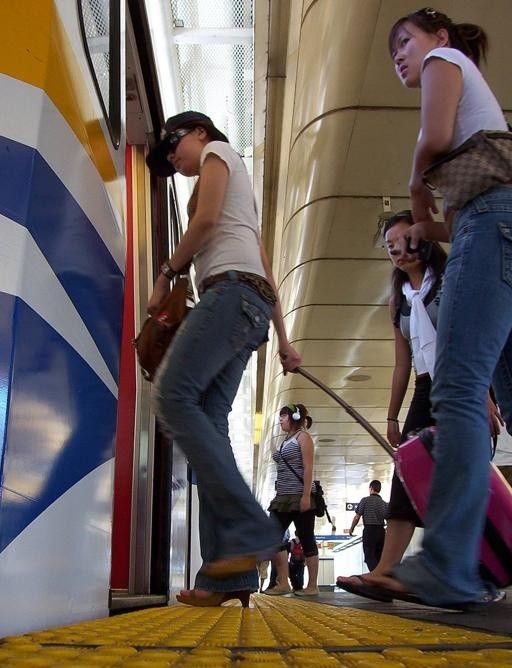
[336,573,397,602]
[357,567,497,612]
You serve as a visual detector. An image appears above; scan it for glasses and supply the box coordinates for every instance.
[379,210,412,239]
[163,125,198,154]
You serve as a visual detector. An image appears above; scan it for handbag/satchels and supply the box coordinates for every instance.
[312,481,326,518]
[421,130,512,211]
[132,278,196,382]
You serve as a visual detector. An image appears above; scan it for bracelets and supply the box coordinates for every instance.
[161,262,176,279]
[386,417,400,422]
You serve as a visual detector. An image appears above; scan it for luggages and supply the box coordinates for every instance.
[280,352,512,589]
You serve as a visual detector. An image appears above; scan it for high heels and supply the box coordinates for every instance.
[176,588,252,609]
[202,537,284,575]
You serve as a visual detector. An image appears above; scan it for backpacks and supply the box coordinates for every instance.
[291,538,306,562]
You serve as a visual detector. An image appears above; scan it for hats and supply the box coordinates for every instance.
[145,111,214,177]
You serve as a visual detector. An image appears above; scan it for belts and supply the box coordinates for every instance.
[443,203,455,236]
[196,271,279,302]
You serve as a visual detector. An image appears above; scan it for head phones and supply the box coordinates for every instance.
[291,406,301,421]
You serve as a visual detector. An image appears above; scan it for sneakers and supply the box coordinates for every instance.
[294,589,320,597]
[265,587,292,596]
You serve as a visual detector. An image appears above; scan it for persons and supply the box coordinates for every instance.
[335,207,505,603]
[256,560,269,592]
[265,403,322,595]
[288,530,305,592]
[350,479,390,572]
[353,6,511,610]
[142,113,302,610]
